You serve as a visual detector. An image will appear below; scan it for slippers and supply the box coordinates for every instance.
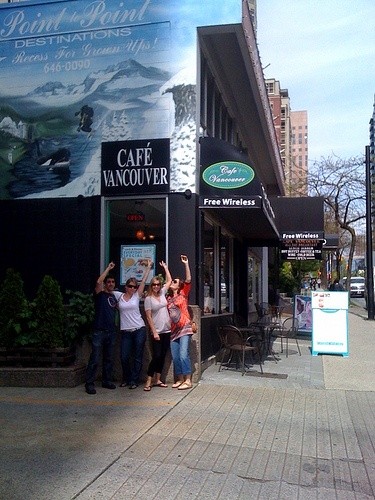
[144,386,151,391]
[154,383,168,387]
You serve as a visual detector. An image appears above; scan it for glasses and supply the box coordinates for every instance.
[128,285,137,289]
[172,279,179,285]
[152,283,161,286]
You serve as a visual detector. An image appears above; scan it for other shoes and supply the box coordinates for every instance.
[172,380,184,388]
[177,383,192,389]
[120,382,127,387]
[129,384,136,389]
[102,382,116,389]
[86,386,96,394]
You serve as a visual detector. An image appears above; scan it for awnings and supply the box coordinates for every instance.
[324,233,339,251]
[199,136,282,246]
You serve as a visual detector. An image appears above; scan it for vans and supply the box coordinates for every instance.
[342,276,365,298]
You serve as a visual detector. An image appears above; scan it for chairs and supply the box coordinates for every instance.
[215,301,302,376]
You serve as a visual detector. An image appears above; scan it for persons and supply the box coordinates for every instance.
[331,278,343,291]
[86,255,192,394]
[310,277,321,290]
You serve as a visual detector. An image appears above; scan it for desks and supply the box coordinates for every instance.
[251,325,281,363]
